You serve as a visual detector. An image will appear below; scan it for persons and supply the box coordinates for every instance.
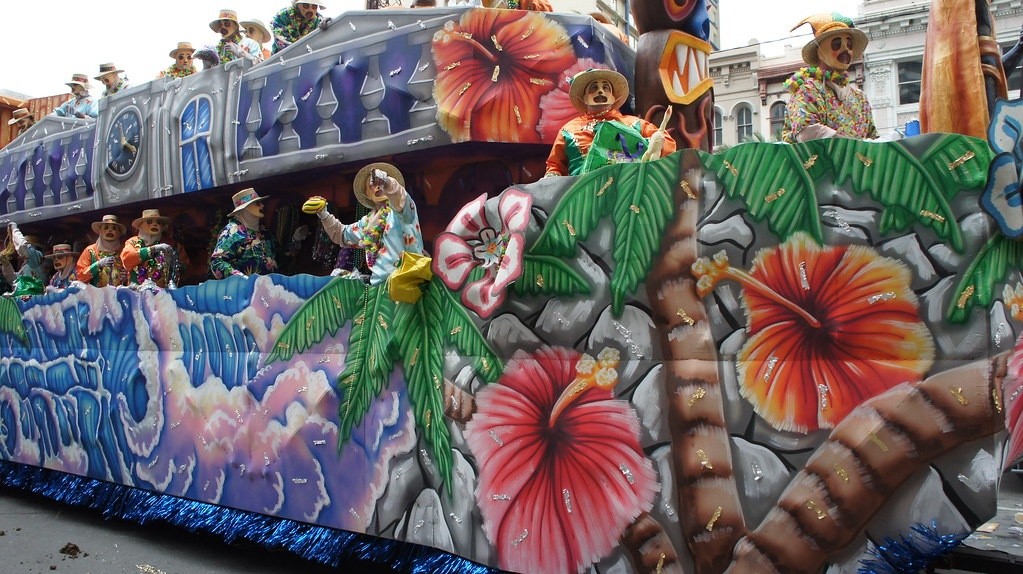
[77,215,130,288]
[269,0,333,55]
[209,9,264,66]
[94,62,131,97]
[239,18,274,58]
[44,244,79,293]
[9,108,37,136]
[121,210,181,289]
[156,42,198,79]
[54,74,98,119]
[783,12,880,142]
[0,221,50,295]
[209,188,280,279]
[543,68,676,179]
[308,163,423,282]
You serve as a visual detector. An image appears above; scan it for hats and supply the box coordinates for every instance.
[353,163,405,208]
[169,41,195,59]
[92,215,126,237]
[24,235,45,249]
[295,0,326,10]
[790,13,869,66]
[93,63,124,80]
[132,209,170,231]
[238,19,271,43]
[227,188,269,216]
[65,74,90,90]
[44,244,80,259]
[209,9,240,34]
[569,68,629,116]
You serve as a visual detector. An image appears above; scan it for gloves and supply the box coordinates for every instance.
[99,256,116,267]
[155,243,172,253]
